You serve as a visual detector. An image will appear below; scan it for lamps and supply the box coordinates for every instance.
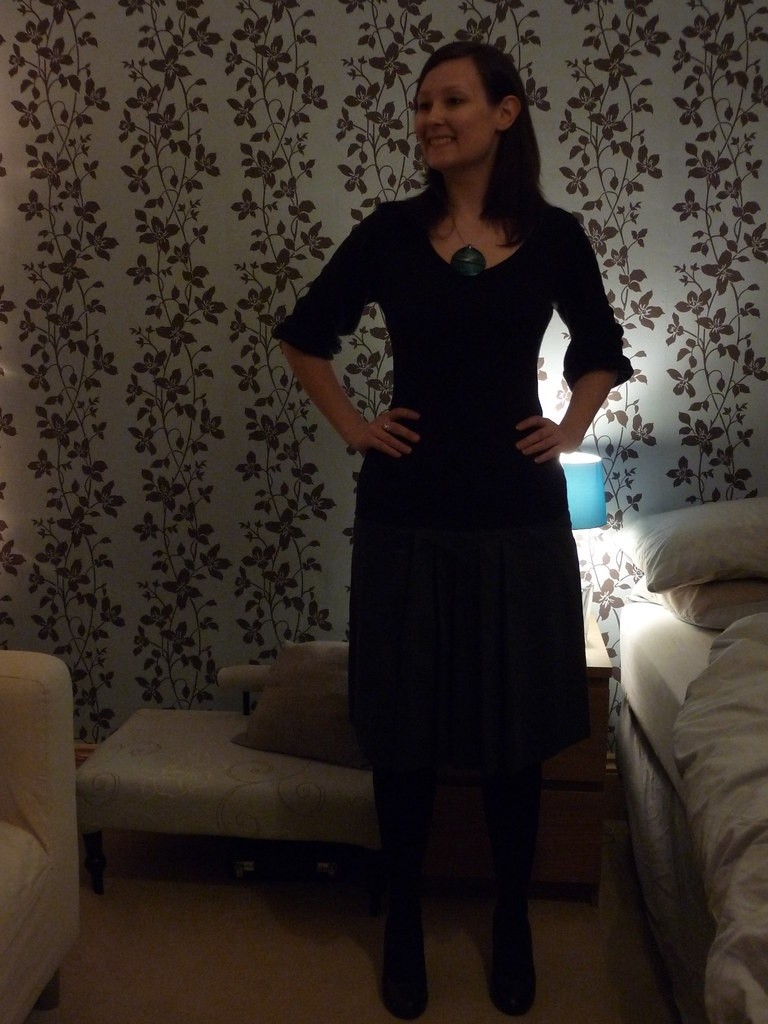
[559,449,607,531]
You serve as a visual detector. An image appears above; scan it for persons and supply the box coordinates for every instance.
[271,42,633,1021]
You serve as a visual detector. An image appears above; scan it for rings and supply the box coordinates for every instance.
[384,420,391,433]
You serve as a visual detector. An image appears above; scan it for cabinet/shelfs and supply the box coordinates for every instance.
[435,611,613,903]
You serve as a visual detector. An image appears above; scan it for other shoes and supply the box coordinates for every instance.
[381,913,428,1021]
[488,912,536,1016]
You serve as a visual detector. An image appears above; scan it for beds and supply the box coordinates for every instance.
[619,598,768,1024]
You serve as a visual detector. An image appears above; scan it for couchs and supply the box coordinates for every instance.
[0,651,80,1024]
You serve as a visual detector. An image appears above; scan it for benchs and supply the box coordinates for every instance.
[75,665,387,916]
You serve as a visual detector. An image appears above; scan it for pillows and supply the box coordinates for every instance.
[636,497,768,594]
[231,639,366,769]
[671,579,768,630]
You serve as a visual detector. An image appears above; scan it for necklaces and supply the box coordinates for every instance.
[447,205,486,276]
